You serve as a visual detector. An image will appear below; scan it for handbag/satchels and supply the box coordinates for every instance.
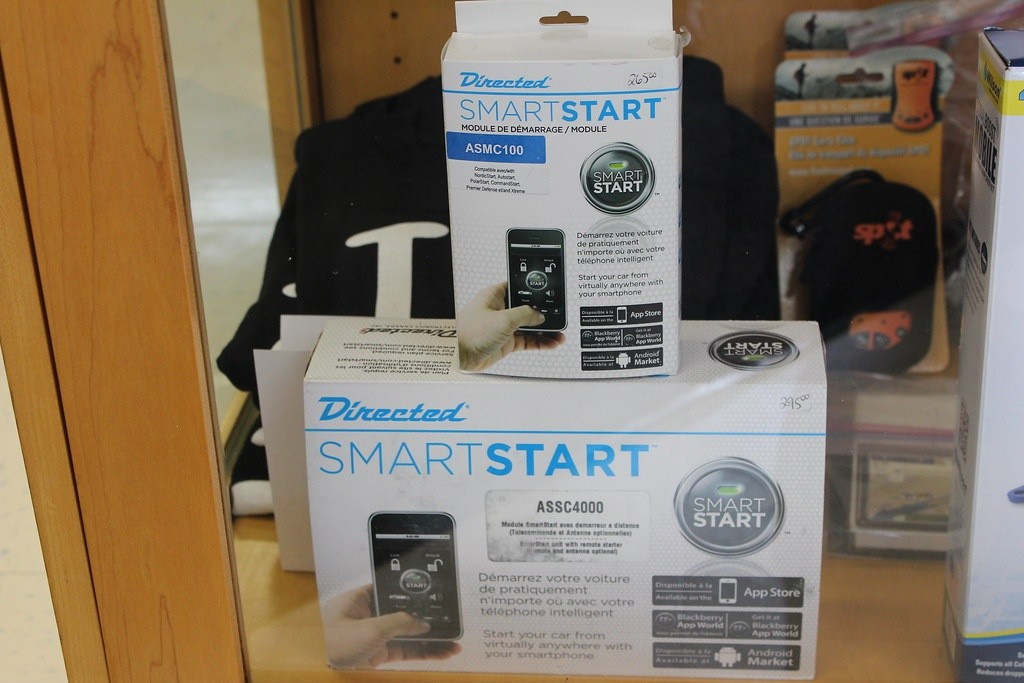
[779,167,937,375]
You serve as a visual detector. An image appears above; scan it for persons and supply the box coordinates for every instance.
[455,282,566,371]
[805,13,817,47]
[794,63,807,96]
[322,583,459,670]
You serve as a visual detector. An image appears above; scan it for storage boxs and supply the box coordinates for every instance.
[300,318,829,681]
[251,315,345,574]
[441,0,684,384]
[935,22,1024,682]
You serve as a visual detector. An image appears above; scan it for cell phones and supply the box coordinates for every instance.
[506,227,568,332]
[368,512,464,641]
